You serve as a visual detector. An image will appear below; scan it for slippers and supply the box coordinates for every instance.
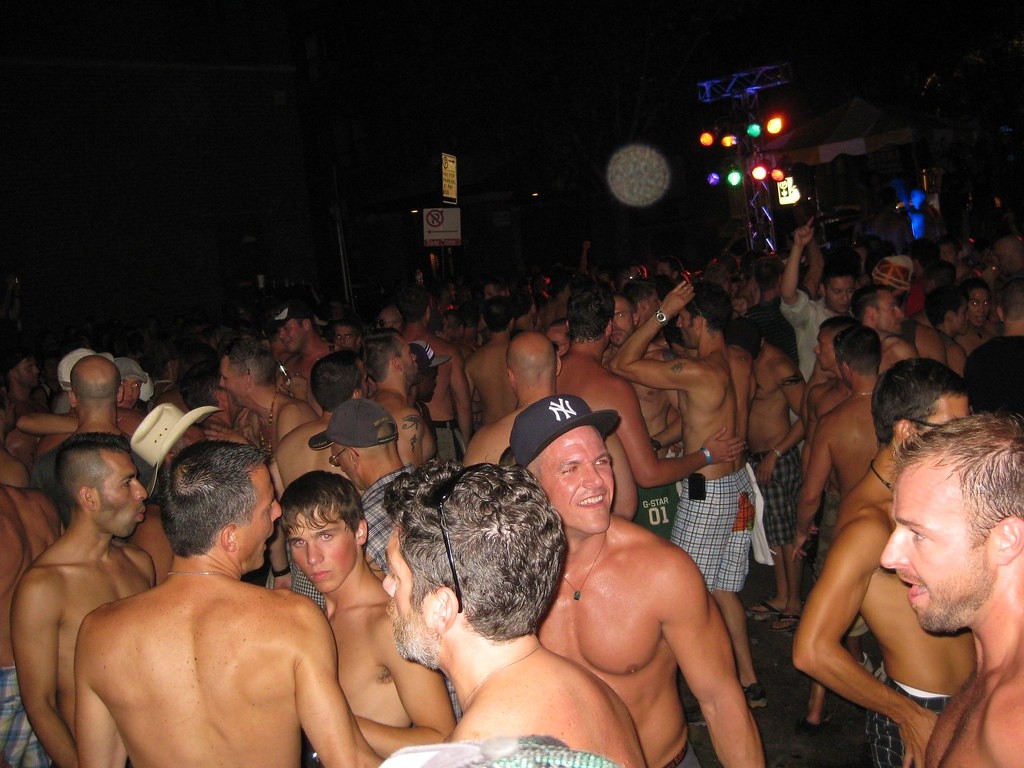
[748,600,784,614]
[769,614,801,632]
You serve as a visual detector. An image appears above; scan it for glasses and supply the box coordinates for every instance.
[553,342,568,352]
[427,463,496,614]
[120,381,141,390]
[615,311,625,318]
[328,447,359,467]
[376,320,398,327]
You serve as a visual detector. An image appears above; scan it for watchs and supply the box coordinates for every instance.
[656,309,668,327]
[651,439,661,451]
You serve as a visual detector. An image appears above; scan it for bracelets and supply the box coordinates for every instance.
[272,565,290,578]
[772,448,781,458]
[700,448,711,465]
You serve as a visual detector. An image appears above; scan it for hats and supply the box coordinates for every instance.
[139,371,154,402]
[405,340,451,373]
[56,348,114,391]
[871,255,913,293]
[268,302,313,327]
[115,357,148,383]
[511,394,618,468]
[315,315,328,326]
[308,398,398,451]
[130,402,224,499]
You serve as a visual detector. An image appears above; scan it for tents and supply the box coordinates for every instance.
[757,95,976,218]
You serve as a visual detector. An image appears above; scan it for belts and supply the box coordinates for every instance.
[434,421,457,428]
[752,447,793,462]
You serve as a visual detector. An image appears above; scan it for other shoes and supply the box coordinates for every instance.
[684,704,707,728]
[798,714,822,733]
[742,681,768,708]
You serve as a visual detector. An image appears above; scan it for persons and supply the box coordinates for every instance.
[0,153,1024,767]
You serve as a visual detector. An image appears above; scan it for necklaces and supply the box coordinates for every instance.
[168,571,224,577]
[870,459,892,490]
[257,388,279,456]
[460,643,542,707]
[855,392,873,397]
[561,532,606,600]
